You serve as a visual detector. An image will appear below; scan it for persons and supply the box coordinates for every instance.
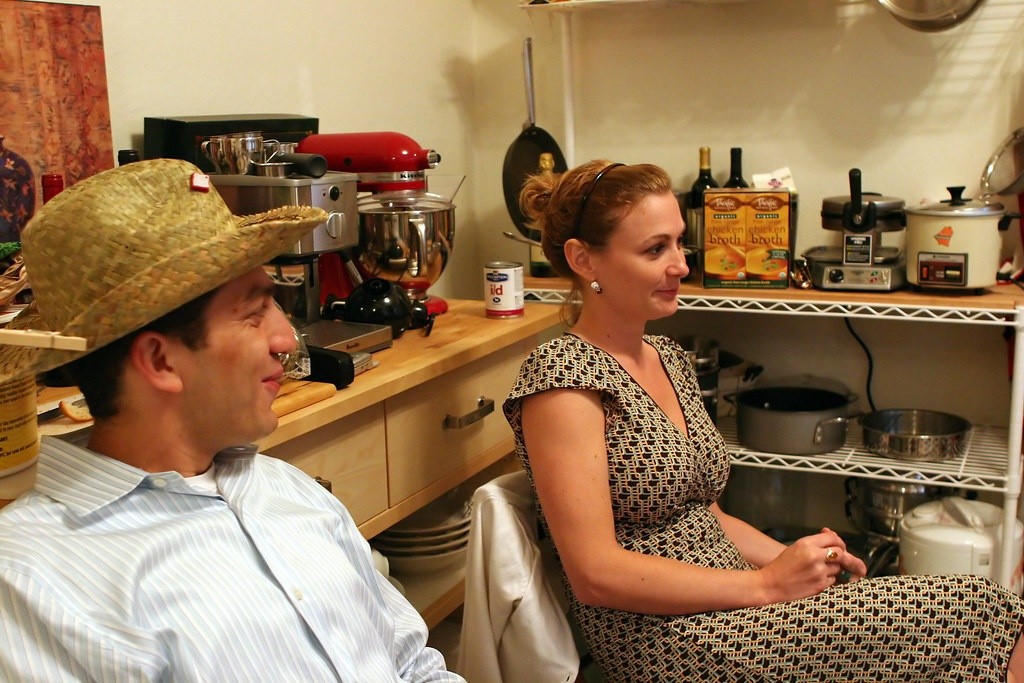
[0,158,468,683]
[503,157,1024,683]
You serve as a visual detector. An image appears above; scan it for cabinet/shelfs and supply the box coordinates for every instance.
[0,297,583,672]
[523,276,1023,592]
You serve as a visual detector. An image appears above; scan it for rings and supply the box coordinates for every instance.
[826,547,838,561]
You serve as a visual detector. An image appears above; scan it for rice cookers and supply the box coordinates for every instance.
[902,126,1024,290]
[898,494,1024,595]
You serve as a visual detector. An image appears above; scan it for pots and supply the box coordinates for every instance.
[721,384,860,454]
[262,142,298,156]
[501,36,570,242]
[843,478,980,550]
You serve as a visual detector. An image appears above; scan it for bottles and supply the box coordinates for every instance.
[684,144,751,247]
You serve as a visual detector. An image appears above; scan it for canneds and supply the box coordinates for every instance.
[482,260,525,319]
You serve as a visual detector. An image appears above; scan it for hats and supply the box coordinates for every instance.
[0,158,331,392]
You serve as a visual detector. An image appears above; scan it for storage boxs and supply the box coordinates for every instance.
[703,187,791,289]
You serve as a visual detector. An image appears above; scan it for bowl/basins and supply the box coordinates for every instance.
[257,162,294,177]
[370,489,472,576]
[857,408,972,461]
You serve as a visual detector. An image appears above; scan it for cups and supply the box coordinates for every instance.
[352,205,455,290]
[201,134,263,175]
